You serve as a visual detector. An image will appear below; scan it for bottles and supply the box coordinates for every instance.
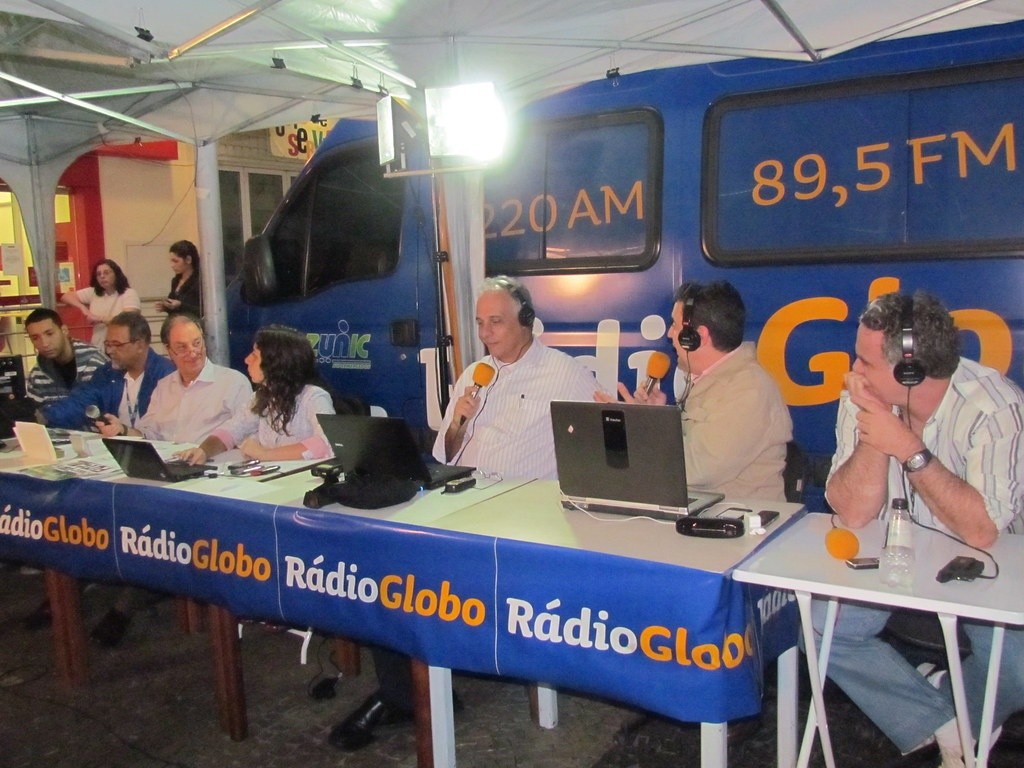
[879,498,915,577]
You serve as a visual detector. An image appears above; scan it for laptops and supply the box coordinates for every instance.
[0,408,71,442]
[315,413,477,489]
[550,401,725,521]
[102,438,218,484]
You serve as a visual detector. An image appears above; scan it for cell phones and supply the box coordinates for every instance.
[227,458,265,475]
[716,507,753,521]
[441,477,476,494]
[846,558,880,569]
[757,510,779,527]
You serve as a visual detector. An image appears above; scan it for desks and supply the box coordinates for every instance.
[1,430,1023,768]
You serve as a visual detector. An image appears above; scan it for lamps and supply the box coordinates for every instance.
[377,80,507,178]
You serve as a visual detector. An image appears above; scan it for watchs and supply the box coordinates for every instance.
[902,449,933,472]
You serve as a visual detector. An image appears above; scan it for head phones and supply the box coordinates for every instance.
[892,295,926,386]
[498,279,535,327]
[677,289,701,352]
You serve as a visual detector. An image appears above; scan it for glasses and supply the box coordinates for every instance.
[103,339,137,350]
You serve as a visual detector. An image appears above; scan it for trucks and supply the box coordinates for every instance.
[200,18,1024,517]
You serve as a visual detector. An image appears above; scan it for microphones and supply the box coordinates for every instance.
[459,363,495,425]
[85,404,110,426]
[826,528,860,560]
[644,352,671,397]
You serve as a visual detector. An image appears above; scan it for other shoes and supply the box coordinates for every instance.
[916,663,953,698]
[24,611,51,630]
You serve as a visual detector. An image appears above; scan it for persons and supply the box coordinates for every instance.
[20,309,106,406]
[59,258,141,356]
[23,312,247,649]
[155,240,200,318]
[327,273,611,747]
[791,288,1024,768]
[597,280,795,747]
[36,308,175,431]
[173,325,337,468]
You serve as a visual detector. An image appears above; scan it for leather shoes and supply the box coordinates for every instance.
[328,692,415,752]
[89,607,131,647]
